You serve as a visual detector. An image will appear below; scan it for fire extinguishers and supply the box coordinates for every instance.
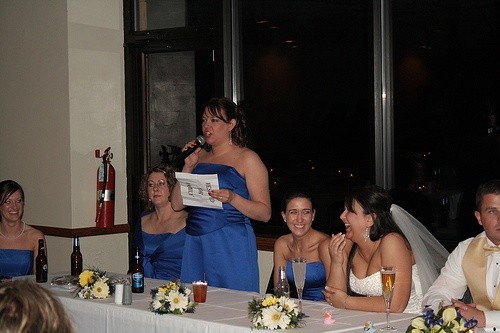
[95,146,116,229]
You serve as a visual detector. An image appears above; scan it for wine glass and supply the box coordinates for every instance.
[292,256,310,320]
[379,265,397,332]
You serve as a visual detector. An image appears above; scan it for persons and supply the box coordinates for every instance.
[140,166,187,281]
[422,183,500,331]
[273,191,332,302]
[0,275,75,333]
[322,187,425,315]
[0,179,47,280]
[171,97,272,293]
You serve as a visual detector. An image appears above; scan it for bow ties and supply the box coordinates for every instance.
[484,244,500,256]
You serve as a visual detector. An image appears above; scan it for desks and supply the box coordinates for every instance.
[13,269,500,333]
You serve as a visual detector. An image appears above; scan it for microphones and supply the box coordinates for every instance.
[179,134,206,160]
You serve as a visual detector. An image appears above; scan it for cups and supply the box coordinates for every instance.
[115,283,123,305]
[192,280,208,303]
[123,281,132,305]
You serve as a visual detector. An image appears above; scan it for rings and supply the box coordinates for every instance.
[330,303,333,306]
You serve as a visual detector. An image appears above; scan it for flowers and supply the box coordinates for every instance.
[150,276,199,316]
[73,265,116,299]
[247,295,305,330]
[405,298,478,333]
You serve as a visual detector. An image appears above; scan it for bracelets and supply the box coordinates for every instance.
[344,295,349,309]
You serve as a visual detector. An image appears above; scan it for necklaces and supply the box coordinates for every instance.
[0,220,25,240]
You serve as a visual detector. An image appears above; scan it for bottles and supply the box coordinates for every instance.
[70,235,82,277]
[131,247,144,293]
[275,266,290,299]
[35,238,48,283]
